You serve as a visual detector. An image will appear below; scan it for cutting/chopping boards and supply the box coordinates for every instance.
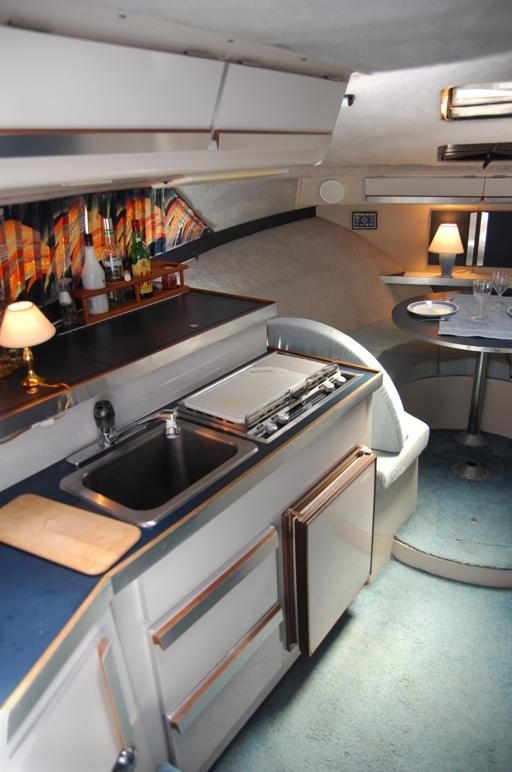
[0,493,141,577]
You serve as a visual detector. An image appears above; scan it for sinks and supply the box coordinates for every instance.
[58,418,261,528]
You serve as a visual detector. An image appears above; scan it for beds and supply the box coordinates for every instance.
[176,214,512,442]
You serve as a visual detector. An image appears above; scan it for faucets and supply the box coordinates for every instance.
[94,399,181,446]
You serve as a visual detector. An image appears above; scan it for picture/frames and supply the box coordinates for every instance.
[351,211,377,230]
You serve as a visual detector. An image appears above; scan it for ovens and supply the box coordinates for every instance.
[282,441,378,659]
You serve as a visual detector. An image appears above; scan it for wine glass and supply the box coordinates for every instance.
[472,271,511,324]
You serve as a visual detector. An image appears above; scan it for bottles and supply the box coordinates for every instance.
[161,266,177,289]
[78,217,153,315]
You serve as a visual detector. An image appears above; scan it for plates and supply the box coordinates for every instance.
[406,300,458,319]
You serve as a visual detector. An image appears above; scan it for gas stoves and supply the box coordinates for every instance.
[176,351,346,436]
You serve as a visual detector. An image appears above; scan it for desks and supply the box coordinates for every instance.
[381,264,512,290]
[389,291,512,450]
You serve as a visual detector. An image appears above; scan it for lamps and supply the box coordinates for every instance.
[427,222,465,276]
[1,301,56,395]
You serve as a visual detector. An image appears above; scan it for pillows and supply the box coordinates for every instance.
[246,259,401,337]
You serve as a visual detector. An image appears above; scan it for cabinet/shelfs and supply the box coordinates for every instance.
[109,399,379,770]
[0,581,166,771]
